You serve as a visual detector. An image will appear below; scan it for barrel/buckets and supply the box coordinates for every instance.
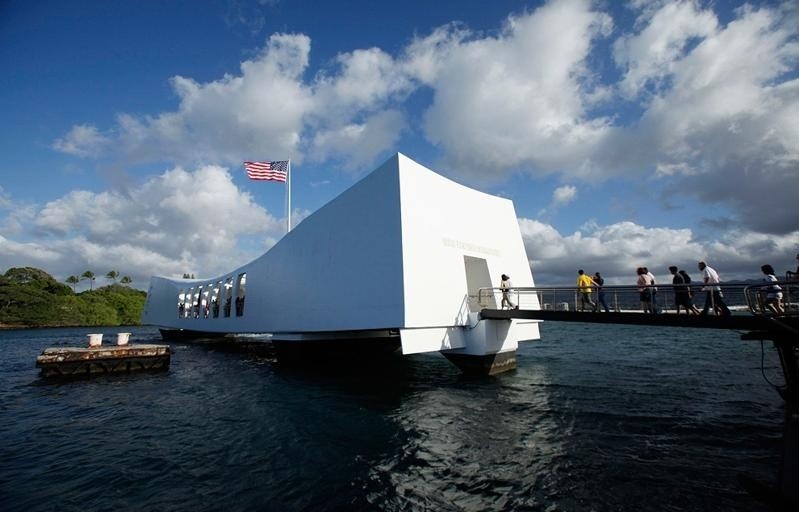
[86,332,104,348]
[117,332,131,346]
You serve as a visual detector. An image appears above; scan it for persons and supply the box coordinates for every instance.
[786,251,799,277]
[754,264,786,315]
[696,260,732,317]
[499,273,518,311]
[679,270,701,315]
[643,267,663,314]
[635,267,653,314]
[576,269,601,312]
[669,265,698,316]
[590,272,610,314]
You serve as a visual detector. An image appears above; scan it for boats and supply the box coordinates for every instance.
[35,343,171,376]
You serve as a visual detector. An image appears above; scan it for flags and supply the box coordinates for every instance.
[241,159,288,183]
